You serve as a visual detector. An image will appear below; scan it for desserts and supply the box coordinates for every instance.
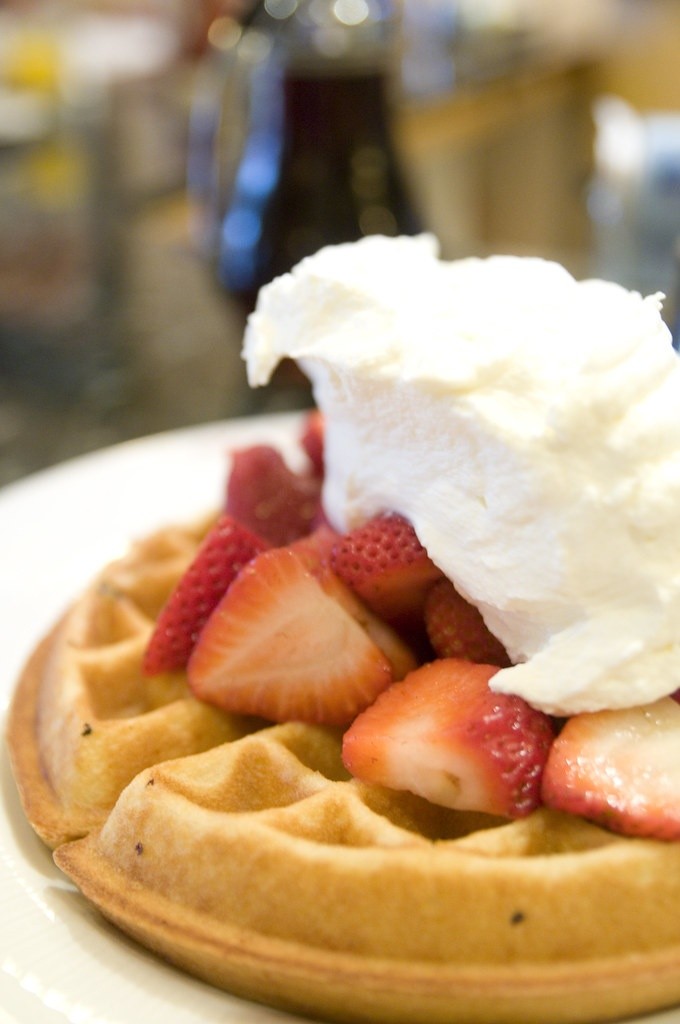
[6,232,680,1024]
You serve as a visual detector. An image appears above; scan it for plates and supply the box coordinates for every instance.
[0,405,680,1024]
